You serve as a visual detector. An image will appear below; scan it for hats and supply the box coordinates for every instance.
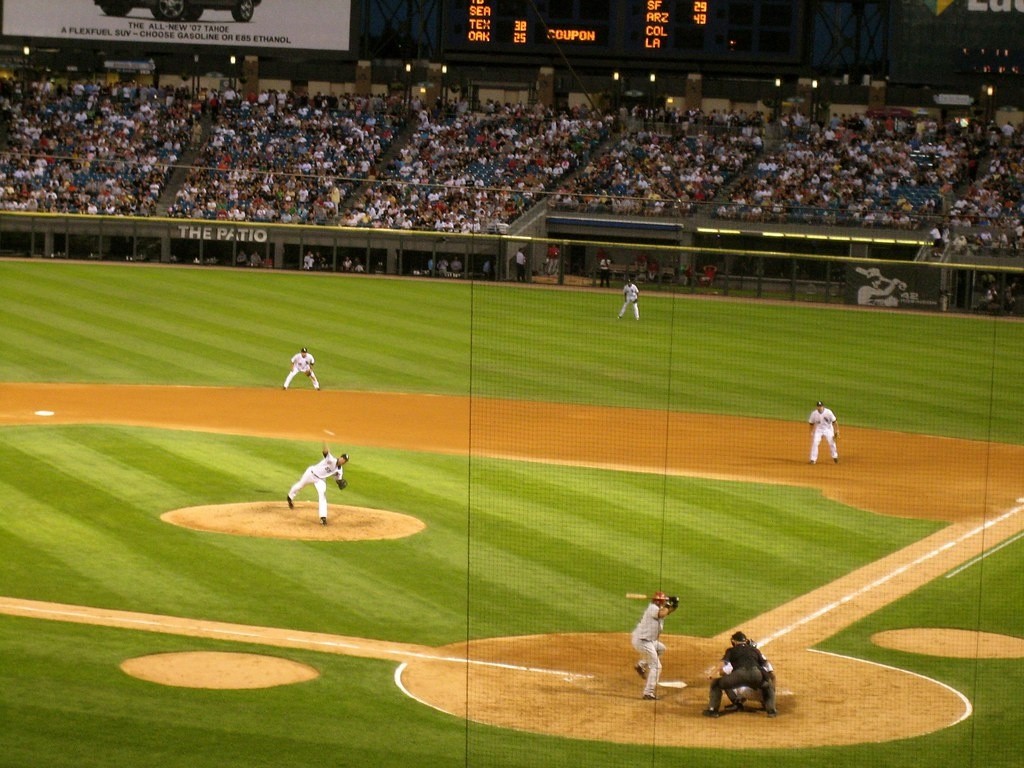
[342,454,349,462]
[301,348,307,353]
[628,279,630,282]
[817,401,823,406]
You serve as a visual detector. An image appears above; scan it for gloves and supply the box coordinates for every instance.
[664,596,679,609]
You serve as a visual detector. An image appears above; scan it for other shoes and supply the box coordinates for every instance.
[702,709,719,718]
[287,496,293,509]
[833,457,838,463]
[635,664,647,679]
[317,388,320,391]
[811,460,816,465]
[642,693,659,700]
[283,387,286,390]
[768,708,777,715]
[321,517,327,525]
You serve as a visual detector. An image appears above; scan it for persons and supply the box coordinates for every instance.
[547,244,560,275]
[375,259,384,274]
[250,251,261,266]
[304,251,328,270]
[428,257,433,276]
[517,248,528,282]
[450,256,462,273]
[343,256,364,272]
[631,596,679,699]
[702,631,777,717]
[808,401,840,464]
[287,446,350,525]
[979,271,1021,316]
[437,256,448,277]
[857,268,907,307]
[282,348,319,389]
[237,250,247,266]
[0,76,1024,258]
[483,256,490,280]
[596,248,718,287]
[617,279,640,321]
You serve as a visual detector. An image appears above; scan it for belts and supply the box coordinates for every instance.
[312,471,320,479]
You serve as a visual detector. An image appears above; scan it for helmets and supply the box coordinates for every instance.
[732,632,747,642]
[747,638,758,649]
[653,590,667,605]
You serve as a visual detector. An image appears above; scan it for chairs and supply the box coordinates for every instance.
[366,116,540,197]
[169,108,398,225]
[714,133,942,224]
[0,98,180,214]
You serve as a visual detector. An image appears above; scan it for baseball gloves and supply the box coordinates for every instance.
[633,299,638,303]
[304,370,311,377]
[336,479,348,490]
[833,432,840,441]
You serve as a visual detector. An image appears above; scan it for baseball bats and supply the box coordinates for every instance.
[626,593,670,601]
